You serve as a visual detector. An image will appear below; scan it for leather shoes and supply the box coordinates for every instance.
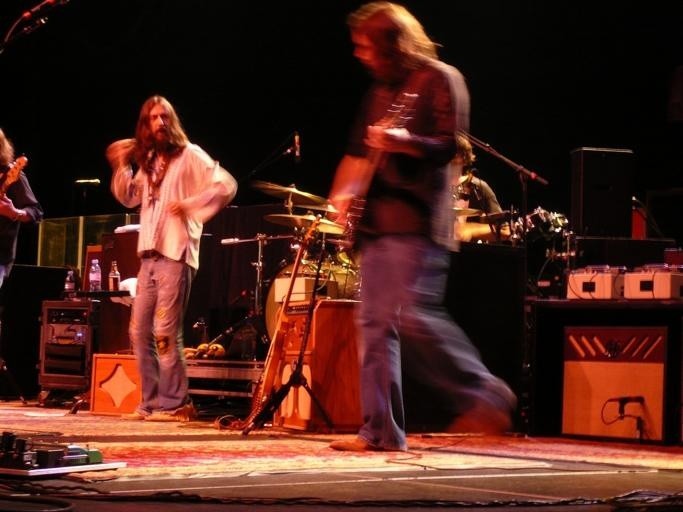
[331,436,407,452]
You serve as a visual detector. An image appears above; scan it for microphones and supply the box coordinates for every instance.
[294,131,304,160]
[76,178,103,186]
[22,0,68,21]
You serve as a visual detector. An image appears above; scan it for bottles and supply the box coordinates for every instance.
[63,270,74,290]
[107,260,121,293]
[629,192,647,240]
[89,258,102,291]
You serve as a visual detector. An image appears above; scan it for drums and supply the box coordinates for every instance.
[267,259,358,341]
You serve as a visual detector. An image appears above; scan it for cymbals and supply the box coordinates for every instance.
[266,215,345,234]
[253,180,328,210]
[452,207,483,218]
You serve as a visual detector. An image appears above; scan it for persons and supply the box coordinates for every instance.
[0,127,42,288]
[328,3,518,453]
[449,127,512,245]
[108,96,238,423]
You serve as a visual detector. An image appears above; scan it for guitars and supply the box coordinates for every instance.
[325,42,438,252]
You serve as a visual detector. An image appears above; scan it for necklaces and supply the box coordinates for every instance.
[145,147,179,201]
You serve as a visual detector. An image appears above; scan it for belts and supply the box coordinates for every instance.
[141,251,163,258]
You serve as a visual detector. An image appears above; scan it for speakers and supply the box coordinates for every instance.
[0,265,79,401]
[565,146,634,237]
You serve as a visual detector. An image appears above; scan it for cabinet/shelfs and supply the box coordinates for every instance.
[39,300,130,407]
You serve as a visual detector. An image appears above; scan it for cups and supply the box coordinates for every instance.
[1,434,16,450]
[16,440,34,451]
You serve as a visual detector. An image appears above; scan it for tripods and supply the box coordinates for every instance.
[194,234,300,361]
[243,249,336,435]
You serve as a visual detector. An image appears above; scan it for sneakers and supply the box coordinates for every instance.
[144,413,189,422]
[121,413,145,420]
